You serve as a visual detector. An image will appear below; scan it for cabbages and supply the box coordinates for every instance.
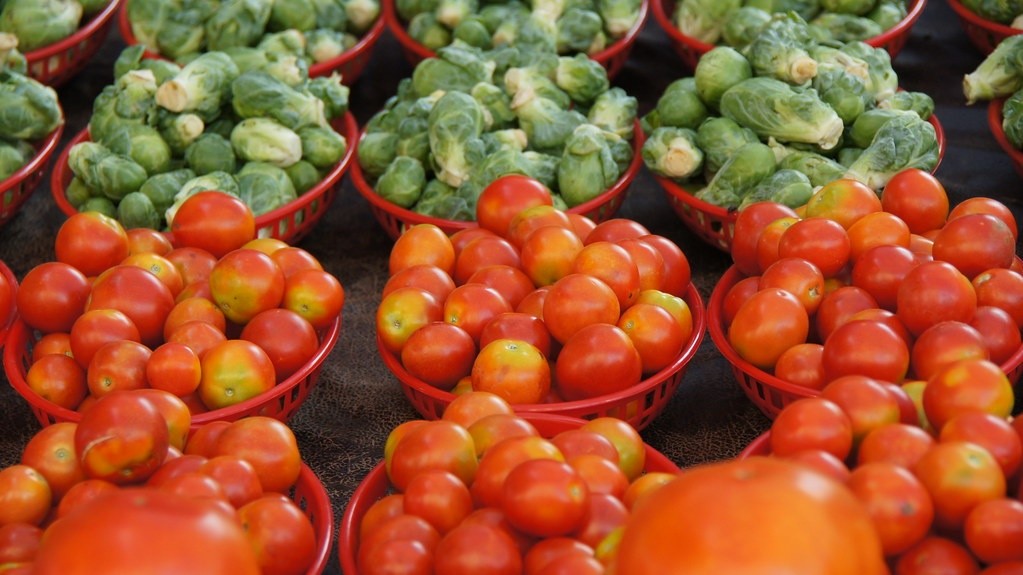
[1,0,1023,227]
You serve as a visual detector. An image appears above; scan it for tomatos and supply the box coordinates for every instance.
[0,167,1023,575]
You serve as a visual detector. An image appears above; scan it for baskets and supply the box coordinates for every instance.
[0,76,63,231]
[4,310,345,431]
[350,115,646,247]
[192,420,334,575]
[652,0,925,71]
[338,414,681,573]
[709,265,1023,425]
[658,86,948,254]
[947,0,1023,52]
[118,0,387,91]
[5,1,121,86]
[988,92,1023,177]
[373,281,708,432]
[380,0,651,87]
[51,106,361,250]
